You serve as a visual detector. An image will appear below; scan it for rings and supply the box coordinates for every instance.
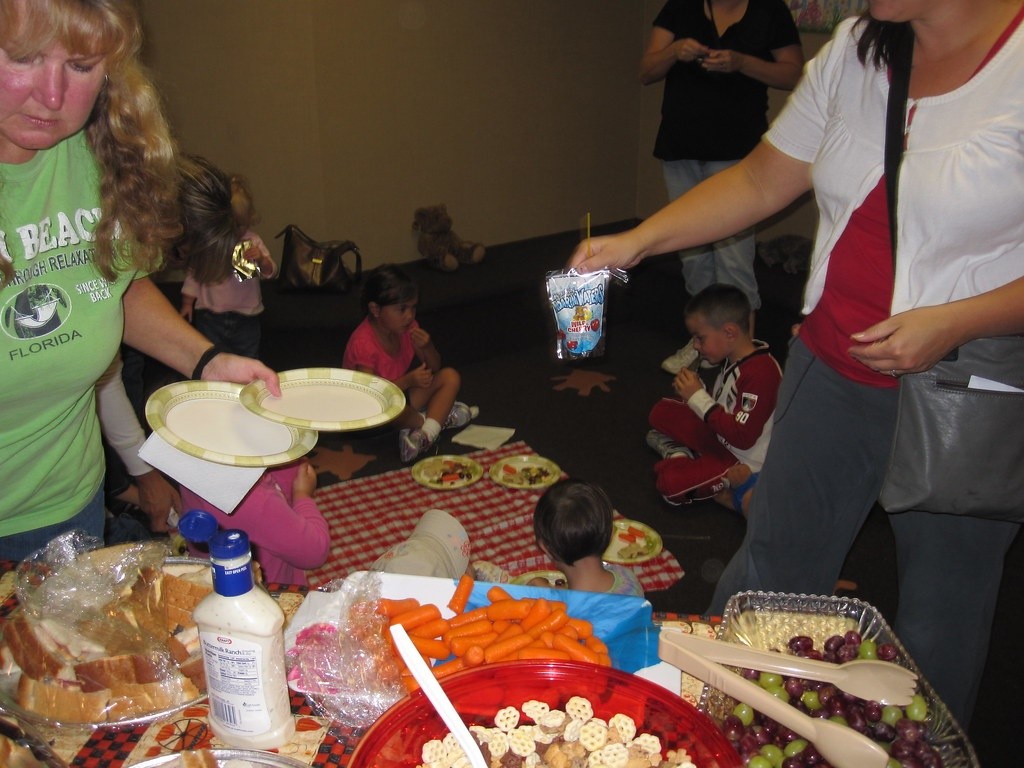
[890,369,898,377]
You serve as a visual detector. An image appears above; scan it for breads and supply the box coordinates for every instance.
[3,563,211,722]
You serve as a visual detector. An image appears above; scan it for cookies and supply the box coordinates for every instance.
[413,697,696,768]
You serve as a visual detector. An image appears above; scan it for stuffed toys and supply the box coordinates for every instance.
[415,204,484,271]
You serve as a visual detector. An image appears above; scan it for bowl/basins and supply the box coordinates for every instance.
[125,748,315,767]
[698,590,980,768]
[0,556,269,768]
[347,659,748,768]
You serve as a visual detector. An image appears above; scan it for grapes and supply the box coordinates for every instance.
[722,630,943,768]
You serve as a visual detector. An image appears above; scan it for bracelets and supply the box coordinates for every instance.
[191,345,226,380]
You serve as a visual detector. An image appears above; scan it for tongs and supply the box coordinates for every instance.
[658,629,918,768]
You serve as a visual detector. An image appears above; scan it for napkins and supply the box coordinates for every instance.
[286,571,457,694]
[451,423,515,450]
[137,432,267,514]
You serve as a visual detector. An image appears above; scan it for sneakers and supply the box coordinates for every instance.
[644,429,696,459]
[440,400,471,432]
[398,428,429,464]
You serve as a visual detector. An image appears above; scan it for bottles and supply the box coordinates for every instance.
[178,509,295,748]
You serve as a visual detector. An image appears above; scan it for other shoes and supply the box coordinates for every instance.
[110,485,146,516]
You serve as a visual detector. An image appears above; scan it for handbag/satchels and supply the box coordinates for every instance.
[278,224,354,297]
[879,335,1022,524]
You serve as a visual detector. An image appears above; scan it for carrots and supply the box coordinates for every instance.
[619,527,645,542]
[503,464,515,473]
[441,474,459,481]
[347,574,611,692]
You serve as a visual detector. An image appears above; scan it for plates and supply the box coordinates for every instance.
[412,455,483,489]
[489,455,560,487]
[239,368,404,431]
[601,519,663,563]
[508,570,568,589]
[145,380,318,467]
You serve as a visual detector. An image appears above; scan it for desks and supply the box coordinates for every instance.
[0,559,722,768]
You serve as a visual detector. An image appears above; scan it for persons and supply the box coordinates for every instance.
[646,283,784,502]
[179,456,330,595]
[563,0,1024,734]
[701,464,899,628]
[98,153,256,534]
[341,264,471,463]
[634,0,805,374]
[474,480,644,599]
[180,176,276,358]
[0,0,282,570]
[369,510,476,580]
